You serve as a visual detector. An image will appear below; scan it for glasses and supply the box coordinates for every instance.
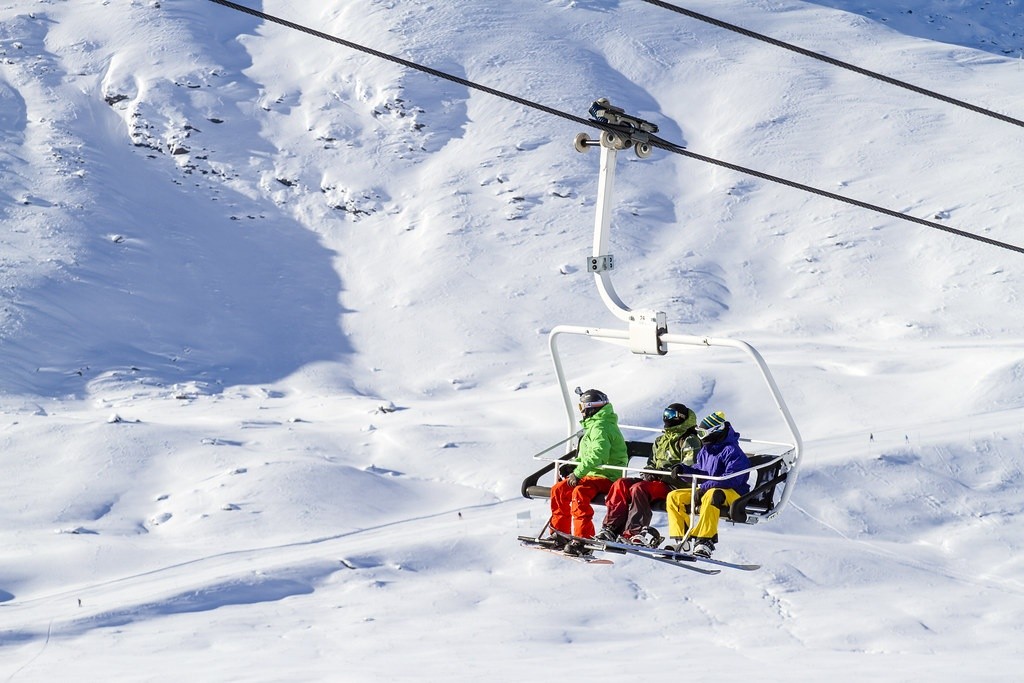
[663,407,687,420]
[579,400,591,413]
[695,425,709,440]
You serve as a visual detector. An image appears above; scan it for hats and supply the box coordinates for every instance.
[700,411,725,436]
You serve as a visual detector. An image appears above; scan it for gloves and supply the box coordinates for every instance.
[671,466,684,480]
[559,464,577,477]
[567,473,579,487]
[694,489,704,505]
[640,467,663,482]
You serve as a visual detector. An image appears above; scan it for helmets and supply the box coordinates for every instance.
[664,404,688,427]
[575,387,607,413]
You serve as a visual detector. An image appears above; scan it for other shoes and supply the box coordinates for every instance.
[564,540,593,555]
[693,538,711,558]
[544,536,569,550]
[616,534,641,551]
[596,530,616,543]
[664,540,682,554]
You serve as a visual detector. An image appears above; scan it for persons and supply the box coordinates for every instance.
[594,403,702,551]
[539,389,629,557]
[664,411,750,558]
